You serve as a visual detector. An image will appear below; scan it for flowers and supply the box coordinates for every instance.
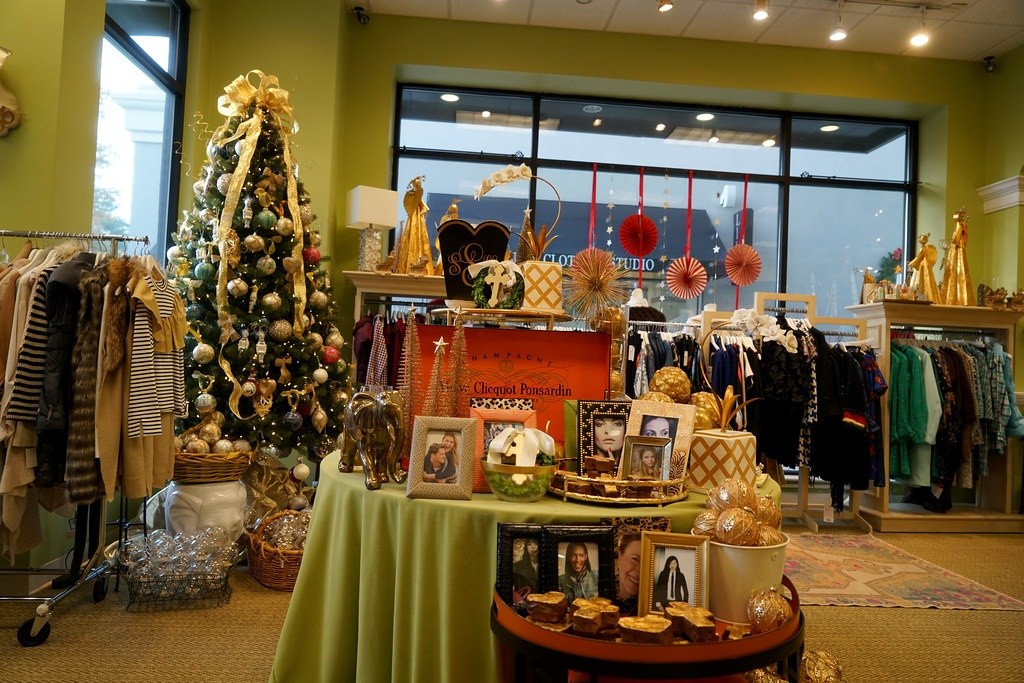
[474,164,561,259]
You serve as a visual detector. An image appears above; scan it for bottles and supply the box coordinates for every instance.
[878,279,907,299]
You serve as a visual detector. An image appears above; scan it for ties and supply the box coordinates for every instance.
[670,573,675,599]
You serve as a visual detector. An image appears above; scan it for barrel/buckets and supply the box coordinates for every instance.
[690,527,790,626]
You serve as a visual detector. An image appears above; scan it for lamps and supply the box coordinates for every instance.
[344,185,399,271]
[0,46,21,138]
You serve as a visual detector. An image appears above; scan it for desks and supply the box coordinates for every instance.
[269,451,781,683]
[490,575,805,683]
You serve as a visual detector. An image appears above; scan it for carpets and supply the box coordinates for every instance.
[780,531,1024,612]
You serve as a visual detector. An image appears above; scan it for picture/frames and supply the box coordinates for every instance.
[578,400,632,476]
[470,408,537,493]
[600,516,671,612]
[405,415,477,501]
[637,530,711,616]
[617,400,697,493]
[495,520,561,616]
[563,400,579,473]
[622,436,673,482]
[539,525,615,605]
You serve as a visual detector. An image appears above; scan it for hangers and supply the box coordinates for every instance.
[0,230,164,296]
[890,327,1012,359]
[364,301,432,325]
[823,330,873,353]
[629,322,761,361]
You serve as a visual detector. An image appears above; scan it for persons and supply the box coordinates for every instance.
[641,416,675,439]
[633,446,661,478]
[655,555,689,611]
[559,543,599,611]
[485,426,503,452]
[513,539,538,596]
[423,432,459,483]
[616,536,642,612]
[393,179,458,274]
[594,418,626,466]
[907,210,976,305]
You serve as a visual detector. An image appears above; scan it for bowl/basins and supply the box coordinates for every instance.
[445,300,477,313]
[479,455,561,503]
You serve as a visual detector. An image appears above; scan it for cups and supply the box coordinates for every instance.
[863,284,877,304]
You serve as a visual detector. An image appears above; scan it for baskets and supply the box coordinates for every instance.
[242,511,310,594]
[171,423,253,483]
